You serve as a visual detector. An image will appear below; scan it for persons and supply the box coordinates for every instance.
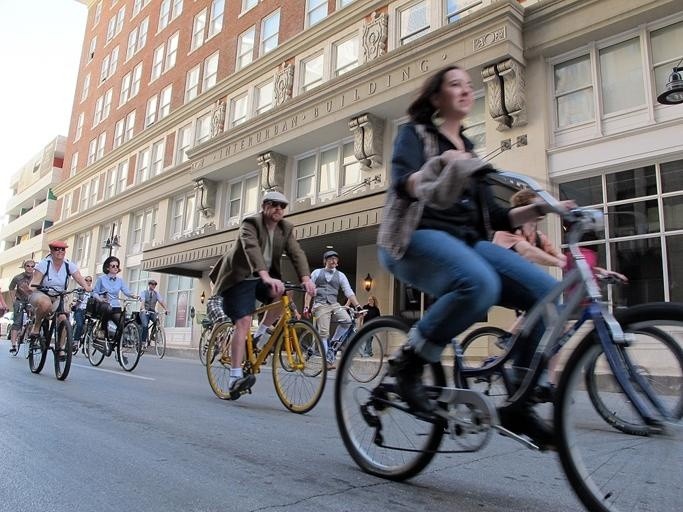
[0,288,7,316]
[492,188,630,402]
[375,66,578,454]
[8,241,170,366]
[204,189,381,401]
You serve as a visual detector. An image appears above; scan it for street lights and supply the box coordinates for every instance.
[654,57,683,105]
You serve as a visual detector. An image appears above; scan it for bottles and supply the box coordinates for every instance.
[324,250,339,258]
[102,255,121,274]
[262,191,289,205]
[49,240,69,249]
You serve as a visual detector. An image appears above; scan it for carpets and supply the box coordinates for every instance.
[150,292,152,299]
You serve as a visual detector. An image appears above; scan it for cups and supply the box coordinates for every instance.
[26,265,35,267]
[266,201,285,209]
[109,265,119,268]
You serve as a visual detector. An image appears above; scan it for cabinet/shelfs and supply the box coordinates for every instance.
[257,325,274,350]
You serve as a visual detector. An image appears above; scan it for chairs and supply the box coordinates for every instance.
[326,363,336,369]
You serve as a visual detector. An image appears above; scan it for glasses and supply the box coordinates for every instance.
[364,274,372,291]
[657,59,683,105]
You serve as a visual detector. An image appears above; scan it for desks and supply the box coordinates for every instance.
[654,57,683,105]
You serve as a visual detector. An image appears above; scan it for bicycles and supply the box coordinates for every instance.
[12,297,167,359]
[294,305,384,384]
[86,298,143,373]
[197,280,328,415]
[453,273,683,435]
[25,284,86,382]
[334,167,679,510]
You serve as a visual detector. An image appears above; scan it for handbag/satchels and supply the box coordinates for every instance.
[534,204,548,218]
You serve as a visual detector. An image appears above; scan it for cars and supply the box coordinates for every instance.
[2,311,13,339]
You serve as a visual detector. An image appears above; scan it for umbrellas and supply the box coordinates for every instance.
[386,343,434,412]
[498,409,554,447]
[230,374,256,400]
